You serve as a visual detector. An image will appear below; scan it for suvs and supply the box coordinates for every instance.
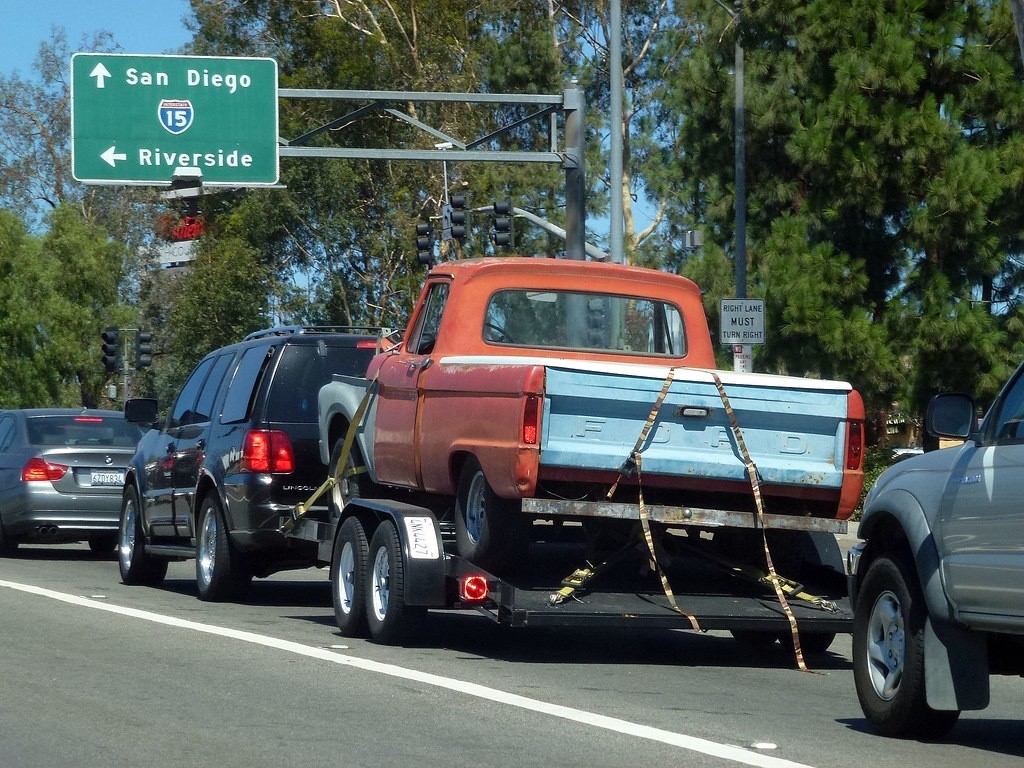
[117,324,406,599]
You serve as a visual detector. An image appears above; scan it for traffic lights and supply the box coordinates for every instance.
[101,326,120,373]
[416,222,434,263]
[135,331,152,371]
[494,196,516,251]
[451,196,470,240]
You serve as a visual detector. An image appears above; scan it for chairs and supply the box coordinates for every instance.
[112,436,134,446]
[41,433,67,444]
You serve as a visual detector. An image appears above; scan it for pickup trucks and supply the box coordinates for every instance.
[318,255,866,574]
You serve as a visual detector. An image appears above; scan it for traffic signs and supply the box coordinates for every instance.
[70,51,279,188]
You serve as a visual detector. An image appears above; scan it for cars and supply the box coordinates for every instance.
[0,408,153,558]
[851,357,1024,736]
